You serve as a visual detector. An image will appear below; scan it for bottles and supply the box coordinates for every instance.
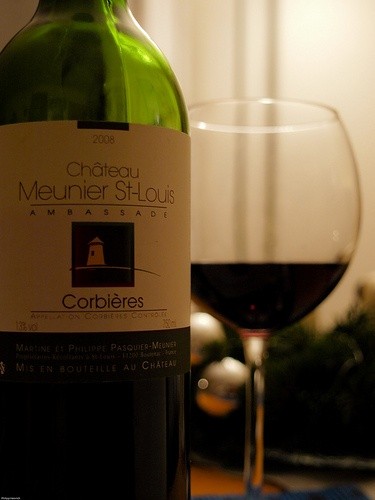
[0,0,193,500]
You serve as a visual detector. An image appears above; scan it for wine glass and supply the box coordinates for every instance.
[186,99,363,500]
